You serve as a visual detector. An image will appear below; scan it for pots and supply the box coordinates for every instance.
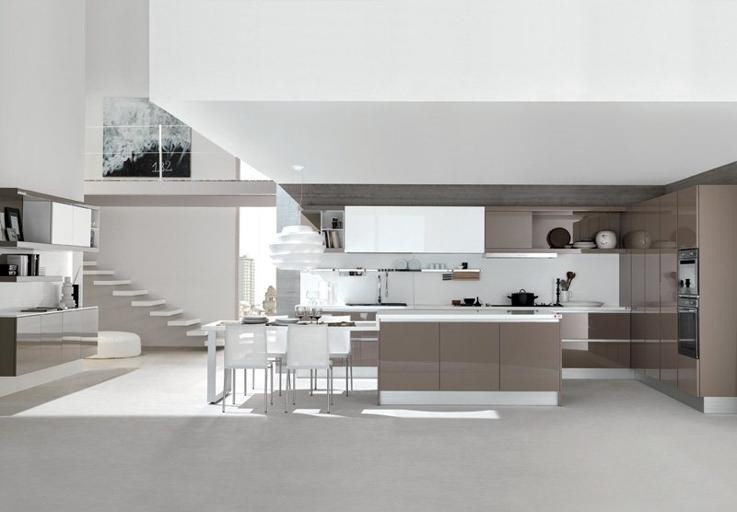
[507,289,539,306]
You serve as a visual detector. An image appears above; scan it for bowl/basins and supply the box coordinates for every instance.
[464,298,475,305]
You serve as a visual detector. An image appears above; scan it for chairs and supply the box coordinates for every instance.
[221,313,352,414]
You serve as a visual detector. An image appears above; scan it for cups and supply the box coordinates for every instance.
[560,290,572,302]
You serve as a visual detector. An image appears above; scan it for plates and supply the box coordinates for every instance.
[408,259,422,269]
[394,257,408,270]
[276,318,299,323]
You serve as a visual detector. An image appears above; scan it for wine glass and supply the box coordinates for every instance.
[295,305,323,324]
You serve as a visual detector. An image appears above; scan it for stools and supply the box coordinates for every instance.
[85,330,143,359]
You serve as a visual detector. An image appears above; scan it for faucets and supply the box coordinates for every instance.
[378,276,382,303]
[553,278,561,306]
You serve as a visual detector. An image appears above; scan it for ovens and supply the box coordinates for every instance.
[676,248,700,359]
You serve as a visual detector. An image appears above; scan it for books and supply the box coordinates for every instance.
[7,253,39,276]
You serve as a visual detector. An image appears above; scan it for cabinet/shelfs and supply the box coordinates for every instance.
[676,356,699,395]
[562,315,627,367]
[319,206,485,255]
[485,210,573,248]
[0,305,99,377]
[618,191,677,388]
[0,185,101,283]
[341,314,378,366]
[676,185,697,250]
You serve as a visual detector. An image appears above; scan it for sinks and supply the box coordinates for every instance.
[559,301,604,307]
[345,303,407,306]
